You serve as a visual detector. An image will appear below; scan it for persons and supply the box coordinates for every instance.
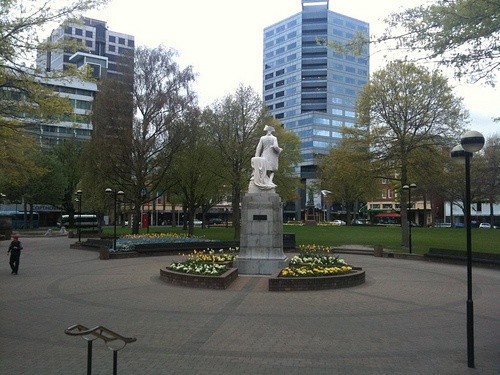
[7,235,23,275]
[253,125,284,181]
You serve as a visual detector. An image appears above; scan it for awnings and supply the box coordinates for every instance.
[365,208,432,213]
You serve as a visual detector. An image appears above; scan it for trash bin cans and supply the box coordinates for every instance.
[283,233,296,249]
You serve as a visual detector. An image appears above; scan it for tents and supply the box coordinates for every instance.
[376,211,402,223]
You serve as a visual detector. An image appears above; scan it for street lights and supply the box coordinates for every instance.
[73,190,84,244]
[400,184,418,253]
[450,129,485,369]
[105,188,126,253]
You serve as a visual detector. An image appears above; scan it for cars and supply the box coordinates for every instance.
[356,219,372,226]
[206,218,222,225]
[432,221,491,230]
[188,218,203,225]
[329,219,344,226]
[124,220,142,226]
[406,221,420,228]
[493,221,499,230]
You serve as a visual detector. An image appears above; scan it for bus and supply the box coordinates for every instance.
[0,210,40,229]
[58,214,100,228]
